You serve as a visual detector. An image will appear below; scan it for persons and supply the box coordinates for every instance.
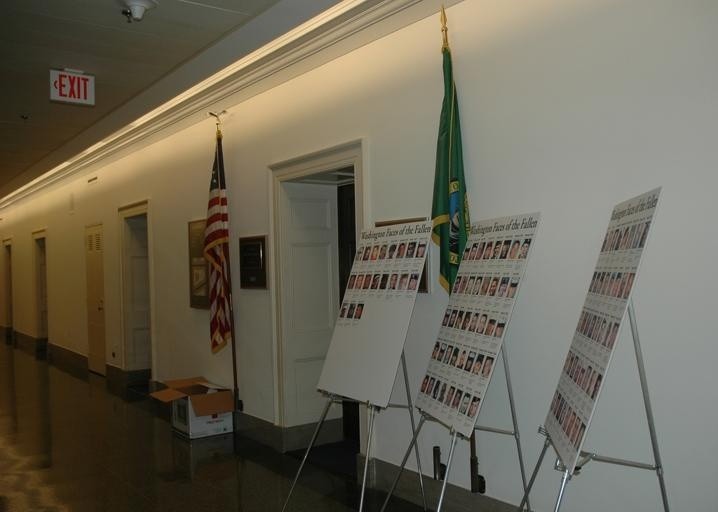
[338,222,426,320]
[418,237,533,421]
[549,219,656,453]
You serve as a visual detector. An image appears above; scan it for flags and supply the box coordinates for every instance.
[203,131,234,353]
[430,46,471,293]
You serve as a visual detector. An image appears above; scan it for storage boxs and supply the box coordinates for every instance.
[148,375,235,439]
